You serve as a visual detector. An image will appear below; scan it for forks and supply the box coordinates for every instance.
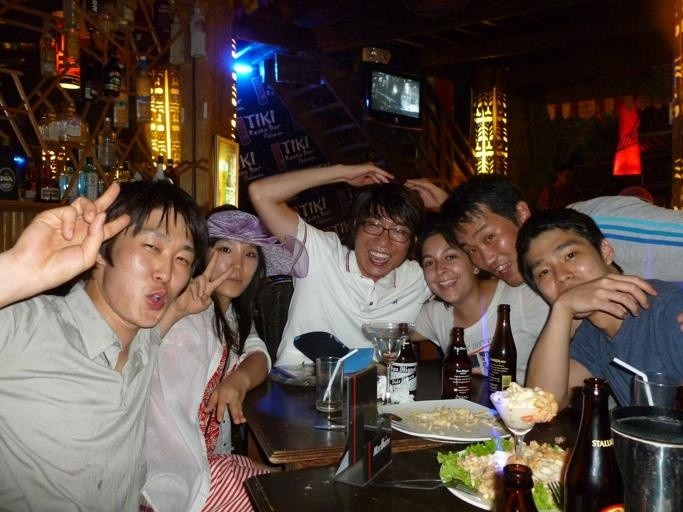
[546,479,565,512]
[394,482,477,496]
[313,417,409,431]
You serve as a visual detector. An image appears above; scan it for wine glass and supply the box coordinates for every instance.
[362,319,416,403]
[491,391,542,459]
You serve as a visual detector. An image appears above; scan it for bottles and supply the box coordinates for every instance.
[102,47,122,95]
[169,9,186,67]
[63,0,82,67]
[493,462,538,512]
[98,118,116,169]
[387,321,419,400]
[135,56,153,128]
[563,377,626,512]
[37,95,88,150]
[113,79,130,129]
[190,6,207,58]
[488,304,517,396]
[440,325,473,401]
[37,21,58,77]
[85,0,134,32]
[16,156,178,204]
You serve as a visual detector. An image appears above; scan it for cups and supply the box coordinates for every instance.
[632,373,683,408]
[314,357,344,415]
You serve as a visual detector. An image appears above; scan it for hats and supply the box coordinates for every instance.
[292,332,375,373]
[205,210,309,278]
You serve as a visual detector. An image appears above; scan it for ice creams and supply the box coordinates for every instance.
[491,382,558,429]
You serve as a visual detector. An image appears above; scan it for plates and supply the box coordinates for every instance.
[265,363,318,387]
[439,449,494,511]
[376,400,512,443]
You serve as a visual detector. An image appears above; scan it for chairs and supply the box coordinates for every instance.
[256,278,299,366]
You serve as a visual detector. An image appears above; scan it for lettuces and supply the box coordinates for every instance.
[437,438,562,512]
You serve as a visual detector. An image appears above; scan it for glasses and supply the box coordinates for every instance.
[355,219,416,243]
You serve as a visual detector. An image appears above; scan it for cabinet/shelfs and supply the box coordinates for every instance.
[0,1,205,209]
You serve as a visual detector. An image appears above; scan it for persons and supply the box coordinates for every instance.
[408,225,551,389]
[247,160,449,370]
[0,176,209,512]
[136,203,280,512]
[512,205,682,412]
[438,172,683,287]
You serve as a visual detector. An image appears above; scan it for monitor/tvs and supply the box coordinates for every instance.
[365,65,426,133]
